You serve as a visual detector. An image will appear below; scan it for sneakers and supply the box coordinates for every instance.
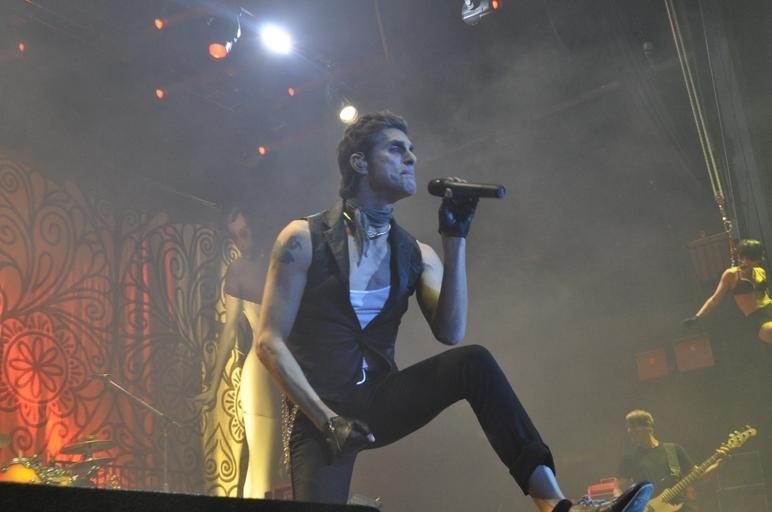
[555,481,652,512]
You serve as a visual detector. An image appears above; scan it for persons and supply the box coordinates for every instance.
[187,201,296,499]
[679,238,771,343]
[616,410,733,511]
[253,110,655,511]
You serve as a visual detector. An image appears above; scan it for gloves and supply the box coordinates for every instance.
[683,317,699,327]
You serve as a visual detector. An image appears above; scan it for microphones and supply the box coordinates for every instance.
[428,178,506,199]
[89,373,110,379]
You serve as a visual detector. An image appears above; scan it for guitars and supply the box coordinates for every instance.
[643,425,758,512]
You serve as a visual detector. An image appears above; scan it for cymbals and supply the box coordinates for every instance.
[68,457,115,468]
[60,439,113,454]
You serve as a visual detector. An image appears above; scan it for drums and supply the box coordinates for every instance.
[0,458,72,487]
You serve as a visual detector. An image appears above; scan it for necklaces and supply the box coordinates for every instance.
[739,268,755,281]
[343,212,393,242]
